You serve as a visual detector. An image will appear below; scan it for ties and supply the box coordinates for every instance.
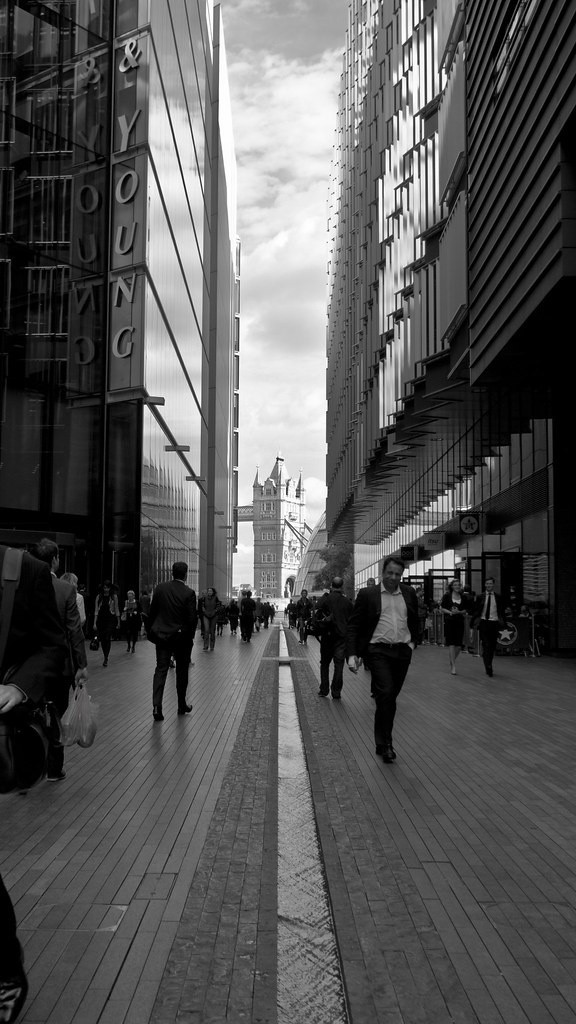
[485,593,491,620]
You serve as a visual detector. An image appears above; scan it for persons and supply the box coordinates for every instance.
[90,580,120,667]
[440,579,474,675]
[346,557,419,763]
[121,590,151,653]
[311,577,352,699]
[414,587,428,645]
[146,561,197,721]
[284,590,319,645]
[474,578,505,677]
[197,586,275,653]
[0,537,101,1024]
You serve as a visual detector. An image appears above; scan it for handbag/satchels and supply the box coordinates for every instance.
[60,678,98,748]
[121,600,128,621]
[0,666,61,796]
[90,631,100,651]
[315,614,336,637]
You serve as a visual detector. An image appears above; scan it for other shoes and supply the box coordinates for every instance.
[318,691,327,696]
[451,668,456,675]
[153,714,164,721]
[210,648,214,651]
[177,705,193,716]
[132,647,135,653]
[127,646,130,651]
[103,661,108,666]
[46,770,66,781]
[203,648,208,650]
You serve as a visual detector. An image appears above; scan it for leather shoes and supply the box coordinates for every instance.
[376,744,396,760]
